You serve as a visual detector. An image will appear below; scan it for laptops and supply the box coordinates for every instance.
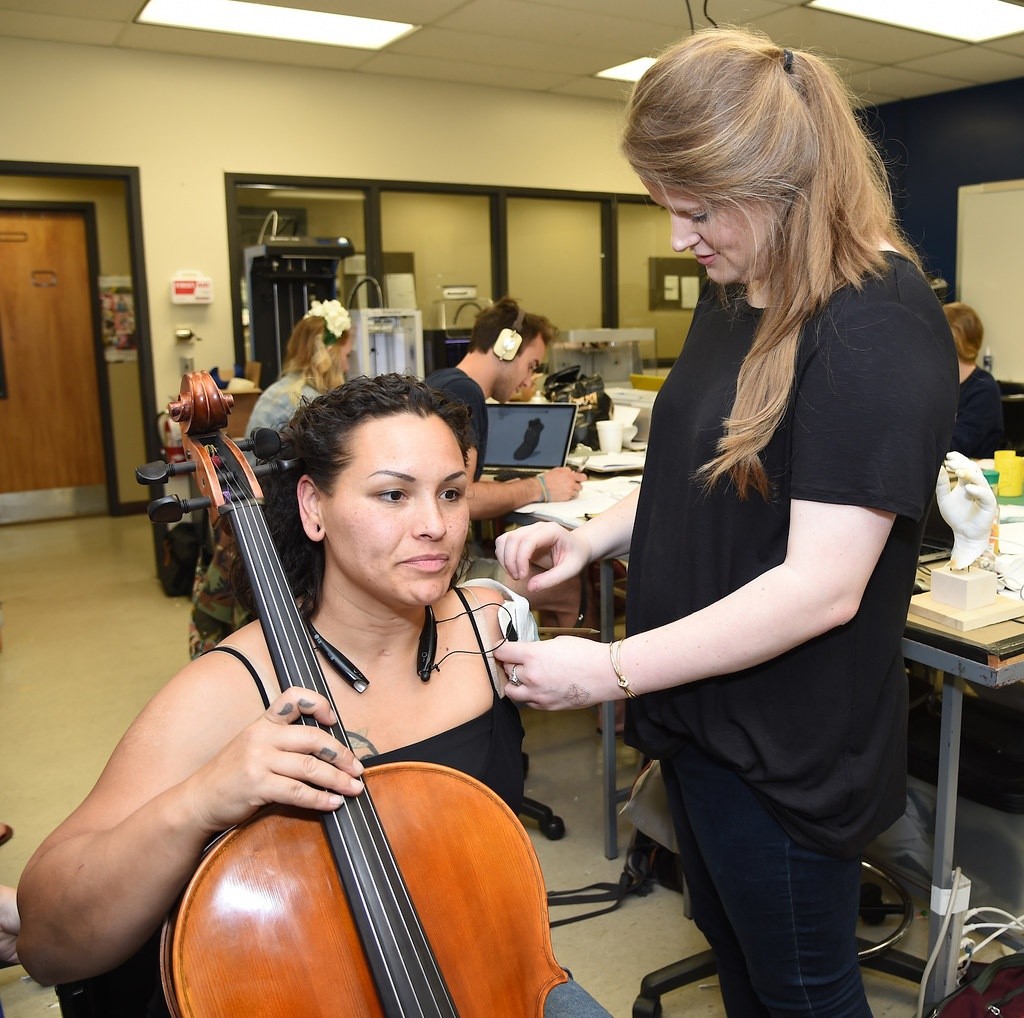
[917,492,955,564]
[478,402,579,484]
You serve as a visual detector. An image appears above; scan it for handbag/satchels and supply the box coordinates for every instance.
[189,518,260,660]
[160,523,204,596]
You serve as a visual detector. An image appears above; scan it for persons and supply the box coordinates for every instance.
[493,30,958,1018]
[16,372,614,1018]
[0,885,21,968]
[241,299,1006,738]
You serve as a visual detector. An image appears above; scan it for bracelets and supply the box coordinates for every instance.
[608,637,639,698]
[536,475,550,503]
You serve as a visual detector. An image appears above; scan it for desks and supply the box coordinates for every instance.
[478,380,1024,1018]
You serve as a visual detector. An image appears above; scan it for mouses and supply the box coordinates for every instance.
[912,578,931,595]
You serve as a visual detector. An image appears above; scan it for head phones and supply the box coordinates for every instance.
[494,309,525,361]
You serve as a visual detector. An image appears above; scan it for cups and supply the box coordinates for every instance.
[596,420,624,455]
[994,450,1024,497]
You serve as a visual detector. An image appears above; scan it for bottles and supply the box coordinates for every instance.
[980,469,999,555]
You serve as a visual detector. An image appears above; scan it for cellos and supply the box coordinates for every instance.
[134,366,571,1017]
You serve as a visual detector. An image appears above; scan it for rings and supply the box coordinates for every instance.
[507,664,521,686]
